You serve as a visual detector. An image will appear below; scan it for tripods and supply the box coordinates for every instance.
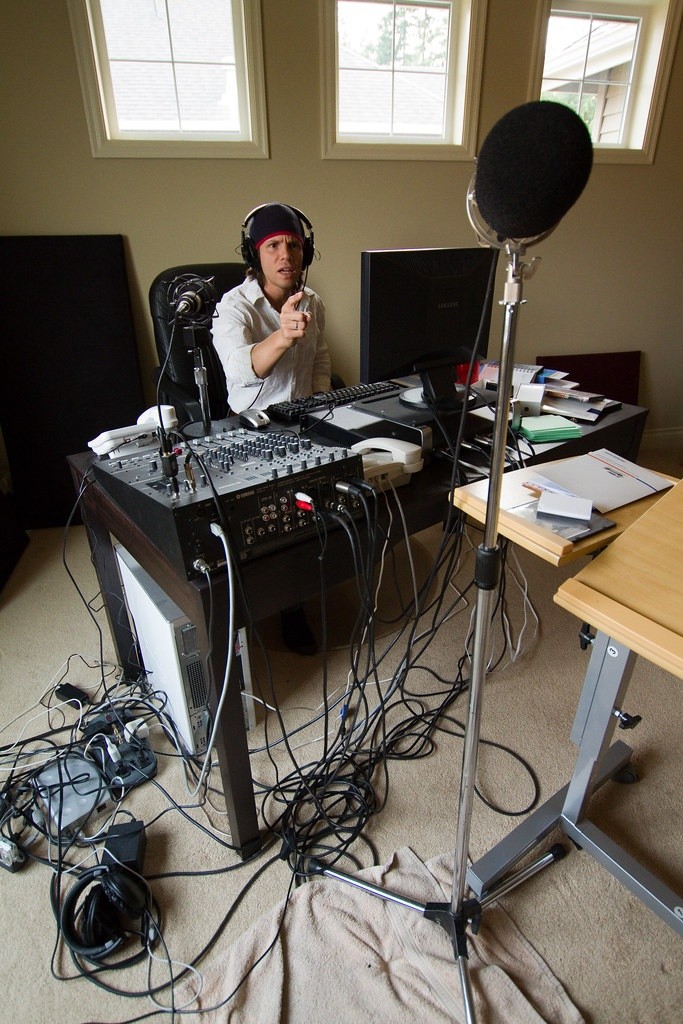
[304,261,568,1024]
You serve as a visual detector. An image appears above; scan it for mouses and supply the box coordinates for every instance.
[237,409,271,429]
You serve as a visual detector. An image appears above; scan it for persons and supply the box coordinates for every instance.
[210,201,331,656]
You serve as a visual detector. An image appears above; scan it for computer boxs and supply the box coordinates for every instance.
[110,541,257,755]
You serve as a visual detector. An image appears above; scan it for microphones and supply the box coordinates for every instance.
[177,276,219,320]
[463,100,596,306]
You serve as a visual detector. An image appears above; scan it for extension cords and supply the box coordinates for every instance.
[86,707,159,791]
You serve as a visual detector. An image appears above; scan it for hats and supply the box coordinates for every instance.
[251,202,306,251]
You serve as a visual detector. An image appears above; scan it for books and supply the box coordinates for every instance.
[544,387,605,402]
[478,362,544,395]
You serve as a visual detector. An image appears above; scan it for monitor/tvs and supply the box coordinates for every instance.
[359,247,519,468]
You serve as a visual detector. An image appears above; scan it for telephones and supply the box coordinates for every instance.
[87,405,178,459]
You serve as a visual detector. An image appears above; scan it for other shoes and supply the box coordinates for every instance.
[281,608,318,656]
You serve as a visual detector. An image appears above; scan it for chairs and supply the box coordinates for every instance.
[148,261,346,423]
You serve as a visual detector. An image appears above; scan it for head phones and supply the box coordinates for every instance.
[59,867,151,961]
[240,203,314,272]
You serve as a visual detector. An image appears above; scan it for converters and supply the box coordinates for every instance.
[54,683,89,709]
[100,819,147,878]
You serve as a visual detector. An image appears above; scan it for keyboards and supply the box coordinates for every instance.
[267,377,415,427]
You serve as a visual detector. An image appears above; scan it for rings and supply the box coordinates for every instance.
[296,322,298,328]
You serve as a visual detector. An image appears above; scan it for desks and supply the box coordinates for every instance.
[65,376,648,855]
[443,462,683,937]
